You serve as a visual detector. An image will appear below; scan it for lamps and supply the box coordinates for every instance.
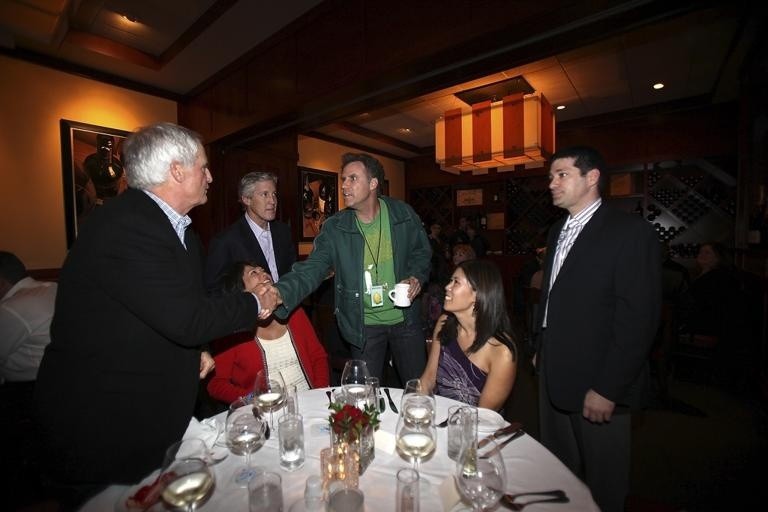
[435,90,556,176]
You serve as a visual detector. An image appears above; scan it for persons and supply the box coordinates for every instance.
[1,248,61,434]
[201,258,331,405]
[450,243,476,267]
[204,169,300,287]
[22,120,284,512]
[426,223,443,246]
[512,249,546,340]
[411,256,519,419]
[259,151,434,388]
[532,145,665,512]
[459,215,476,241]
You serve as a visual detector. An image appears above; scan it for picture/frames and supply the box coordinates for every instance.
[59,119,136,249]
[298,166,338,242]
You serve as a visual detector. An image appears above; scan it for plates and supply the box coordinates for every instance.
[472,406,505,432]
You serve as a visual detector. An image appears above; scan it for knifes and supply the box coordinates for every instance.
[473,422,521,450]
[385,387,398,415]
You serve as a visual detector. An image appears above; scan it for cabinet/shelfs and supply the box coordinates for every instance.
[405,103,738,308]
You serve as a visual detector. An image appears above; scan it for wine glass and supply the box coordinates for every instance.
[394,409,437,495]
[225,399,267,485]
[342,359,371,408]
[402,381,435,428]
[456,435,505,511]
[252,368,287,438]
[161,439,215,512]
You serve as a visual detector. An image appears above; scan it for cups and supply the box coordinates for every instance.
[388,283,411,308]
[325,482,365,512]
[365,378,380,417]
[278,413,305,471]
[397,468,419,512]
[247,472,283,511]
[282,383,298,415]
[448,406,478,460]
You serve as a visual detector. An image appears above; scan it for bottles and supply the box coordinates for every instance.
[305,476,325,507]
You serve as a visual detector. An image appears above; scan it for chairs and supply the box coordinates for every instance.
[504,365,539,439]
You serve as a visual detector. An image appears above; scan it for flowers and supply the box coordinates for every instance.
[327,393,382,449]
